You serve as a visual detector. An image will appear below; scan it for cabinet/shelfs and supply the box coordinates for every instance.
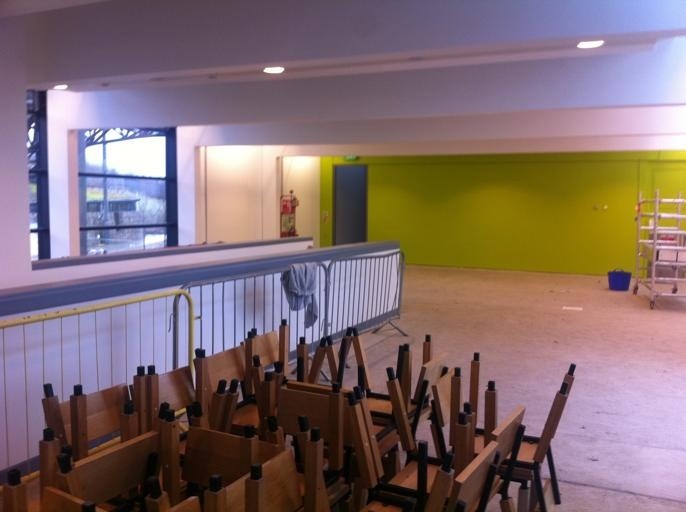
[632,189,686,310]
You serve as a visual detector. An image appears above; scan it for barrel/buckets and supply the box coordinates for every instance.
[607,267,632,291]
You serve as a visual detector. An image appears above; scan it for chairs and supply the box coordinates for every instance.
[0,318,577,512]
